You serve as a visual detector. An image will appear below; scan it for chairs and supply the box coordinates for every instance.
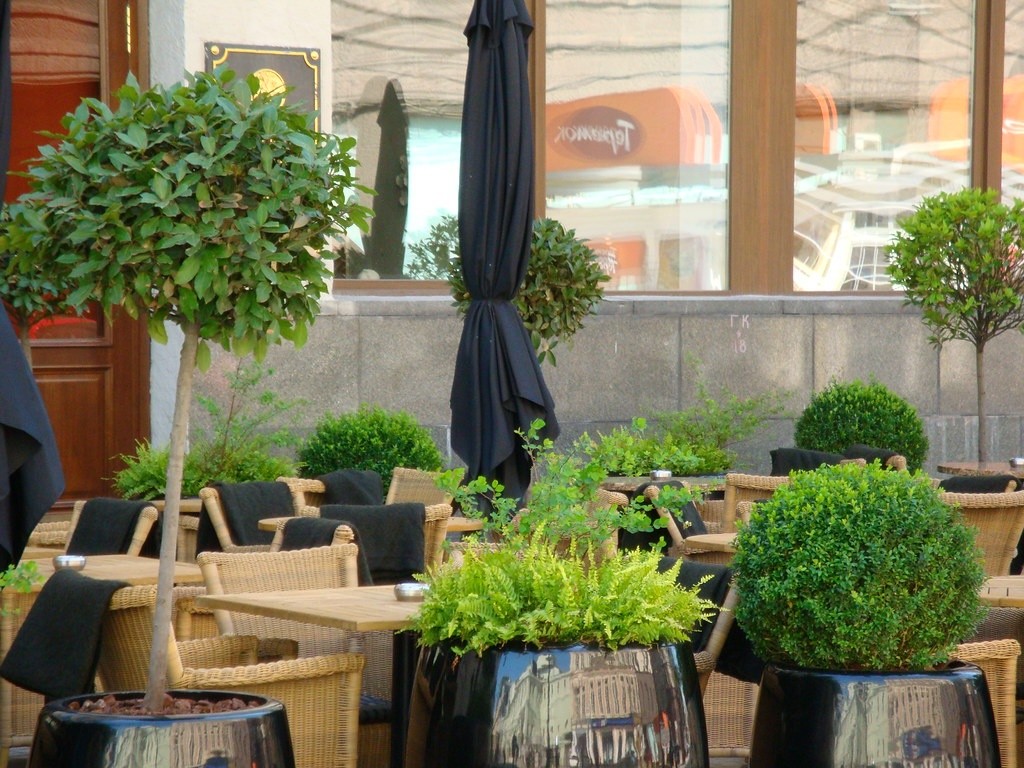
[0,468,1024,768]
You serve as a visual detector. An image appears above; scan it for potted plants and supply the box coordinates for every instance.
[729,458,1002,768]
[392,521,711,768]
[0,63,379,768]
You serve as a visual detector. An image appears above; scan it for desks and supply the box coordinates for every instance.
[148,497,203,513]
[937,461,1024,480]
[605,477,726,491]
[201,585,430,768]
[683,531,741,555]
[254,513,483,532]
[17,553,204,641]
